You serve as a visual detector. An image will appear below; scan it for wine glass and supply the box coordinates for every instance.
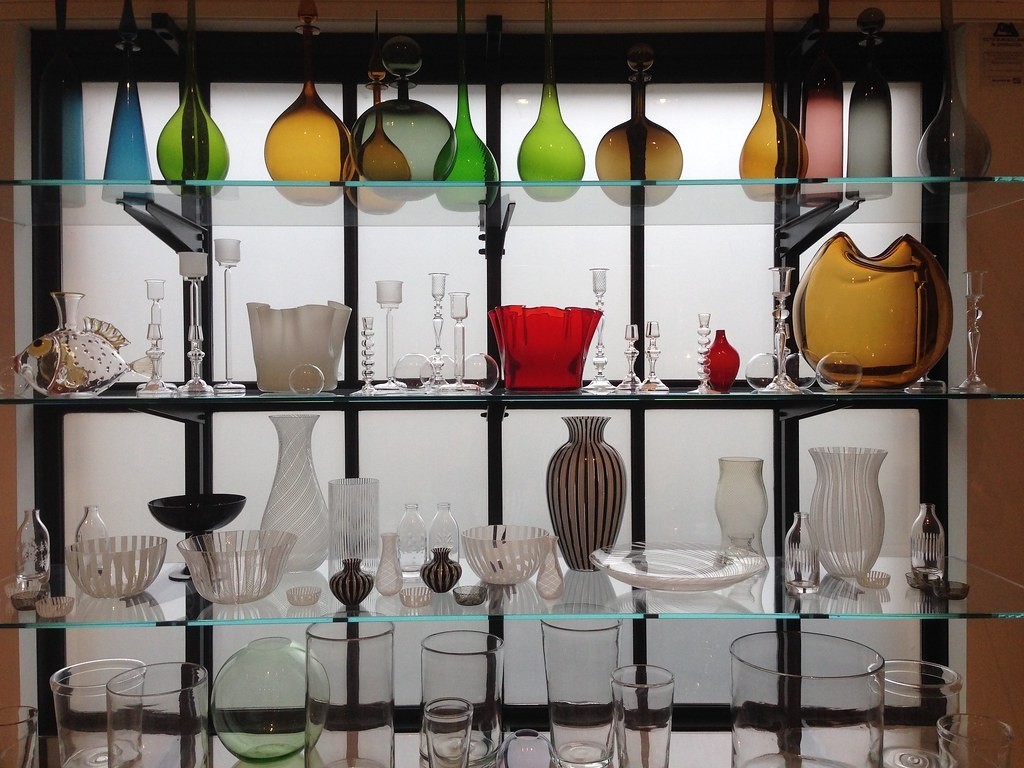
[949,269,1000,392]
[136,238,246,396]
[349,272,482,395]
[582,267,812,394]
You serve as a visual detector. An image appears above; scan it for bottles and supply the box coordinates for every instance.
[704,329,740,393]
[257,415,329,573]
[329,503,459,607]
[213,635,332,764]
[790,231,953,394]
[536,415,627,600]
[736,0,992,202]
[714,447,889,595]
[92,1,683,213]
[911,503,945,581]
[75,505,109,543]
[14,508,51,584]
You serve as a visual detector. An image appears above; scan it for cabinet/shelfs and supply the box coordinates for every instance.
[0,170,1024,768]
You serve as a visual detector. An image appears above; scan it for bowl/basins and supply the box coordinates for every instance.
[246,300,352,395]
[488,305,603,394]
[62,535,168,599]
[177,529,297,605]
[459,524,551,584]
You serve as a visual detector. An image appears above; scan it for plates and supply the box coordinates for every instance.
[587,539,769,592]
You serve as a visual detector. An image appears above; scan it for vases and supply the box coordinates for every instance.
[57,0,987,768]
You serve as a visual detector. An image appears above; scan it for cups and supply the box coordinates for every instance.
[0,656,210,768]
[728,630,1014,768]
[303,622,675,768]
